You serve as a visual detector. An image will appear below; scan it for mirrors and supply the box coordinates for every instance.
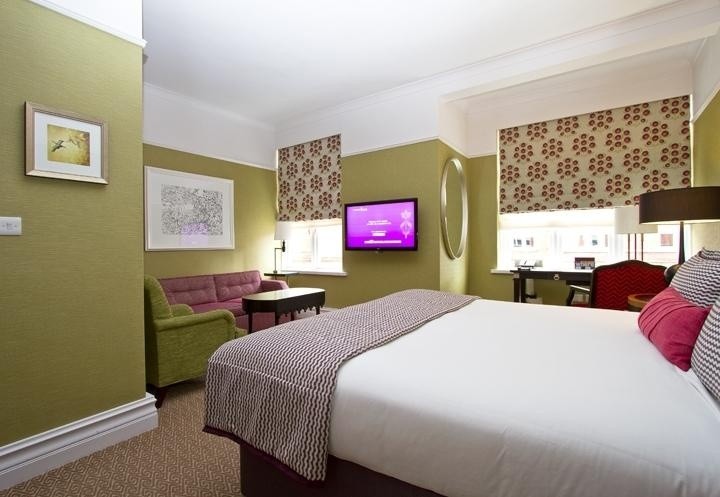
[439,156,470,260]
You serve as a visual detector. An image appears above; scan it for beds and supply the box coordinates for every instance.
[203,287,720,496]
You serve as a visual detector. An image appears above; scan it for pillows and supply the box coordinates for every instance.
[672,242,720,309]
[638,284,712,374]
[690,294,720,402]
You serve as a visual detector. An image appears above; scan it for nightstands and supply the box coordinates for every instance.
[627,293,658,310]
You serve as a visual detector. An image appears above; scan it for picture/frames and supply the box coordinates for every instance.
[23,99,112,186]
[143,163,236,251]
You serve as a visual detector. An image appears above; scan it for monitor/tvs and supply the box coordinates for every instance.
[345,197,418,251]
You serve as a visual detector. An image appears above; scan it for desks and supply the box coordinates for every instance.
[490,265,594,303]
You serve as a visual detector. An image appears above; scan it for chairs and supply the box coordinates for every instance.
[144,272,249,411]
[565,256,670,306]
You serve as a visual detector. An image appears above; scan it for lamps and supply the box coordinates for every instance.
[636,184,720,282]
[614,204,658,263]
[273,220,295,273]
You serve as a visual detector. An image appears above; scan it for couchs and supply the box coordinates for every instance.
[156,268,295,334]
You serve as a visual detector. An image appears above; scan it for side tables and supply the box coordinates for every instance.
[263,270,298,284]
